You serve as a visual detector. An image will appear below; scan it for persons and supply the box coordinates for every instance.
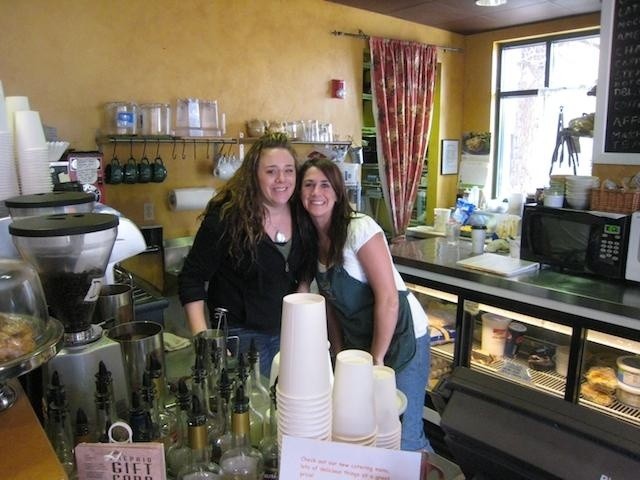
[288,159,435,456]
[176,131,302,380]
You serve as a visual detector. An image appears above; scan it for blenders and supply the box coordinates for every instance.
[9,213,129,431]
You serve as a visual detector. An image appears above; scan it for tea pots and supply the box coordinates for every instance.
[263,119,287,132]
[214,156,242,182]
[245,117,268,137]
[102,157,168,184]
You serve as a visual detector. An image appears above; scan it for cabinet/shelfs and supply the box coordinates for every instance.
[362,60,429,175]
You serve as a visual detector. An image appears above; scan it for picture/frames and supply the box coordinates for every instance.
[441,139,460,177]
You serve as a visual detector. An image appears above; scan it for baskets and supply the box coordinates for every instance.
[591,178,640,211]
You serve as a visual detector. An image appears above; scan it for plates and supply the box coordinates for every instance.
[462,136,489,155]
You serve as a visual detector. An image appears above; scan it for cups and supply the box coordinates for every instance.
[447,222,460,244]
[470,223,487,254]
[431,208,451,233]
[552,345,571,376]
[542,184,563,207]
[284,117,332,142]
[0,79,55,204]
[480,311,510,357]
[506,323,528,358]
[274,291,403,462]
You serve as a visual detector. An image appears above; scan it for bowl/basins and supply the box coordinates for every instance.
[511,191,523,214]
[562,174,599,211]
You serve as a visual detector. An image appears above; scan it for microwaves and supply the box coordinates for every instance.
[519,201,627,281]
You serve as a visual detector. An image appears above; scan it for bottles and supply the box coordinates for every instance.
[41,337,277,480]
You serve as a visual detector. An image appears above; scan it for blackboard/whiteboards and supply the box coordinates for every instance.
[592,0,640,166]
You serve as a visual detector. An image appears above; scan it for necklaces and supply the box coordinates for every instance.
[266,210,288,245]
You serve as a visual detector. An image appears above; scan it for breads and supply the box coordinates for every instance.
[580,364,618,407]
[0,316,35,359]
[471,349,498,366]
